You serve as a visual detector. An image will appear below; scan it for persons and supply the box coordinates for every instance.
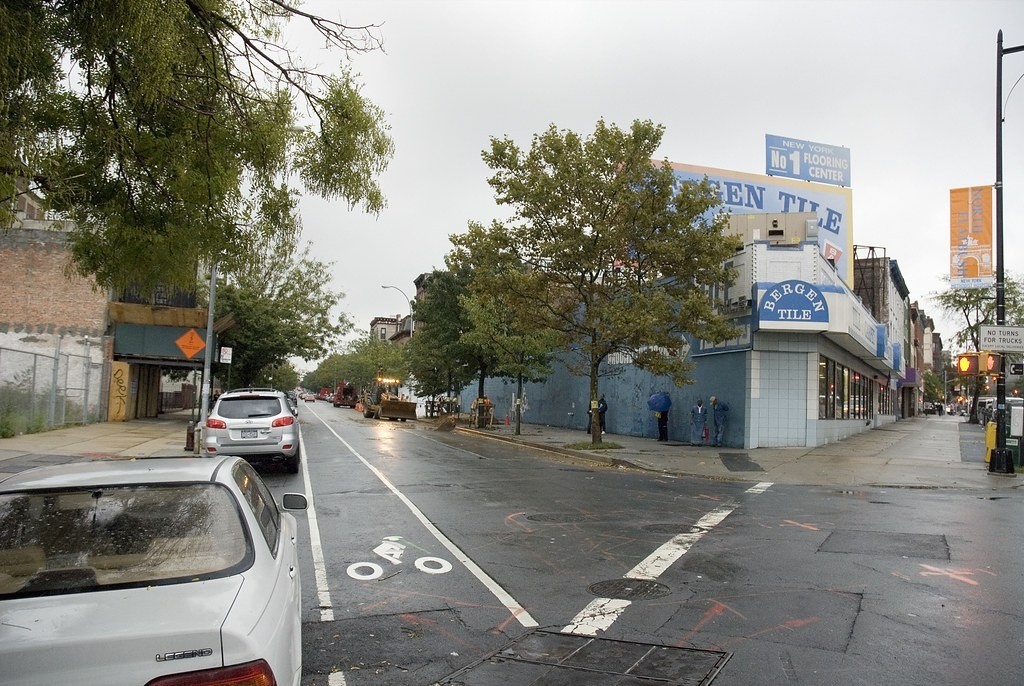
[709,396,729,447]
[657,411,669,442]
[214,390,221,401]
[471,395,490,411]
[586,393,608,434]
[938,403,943,416]
[689,399,708,447]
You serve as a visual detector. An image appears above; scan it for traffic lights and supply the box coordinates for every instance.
[957,352,1001,377]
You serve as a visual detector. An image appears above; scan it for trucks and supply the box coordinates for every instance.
[332,383,358,408]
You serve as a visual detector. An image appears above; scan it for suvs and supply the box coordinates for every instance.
[206,387,300,473]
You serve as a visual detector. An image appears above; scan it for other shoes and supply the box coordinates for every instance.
[601,431,605,434]
[690,444,694,446]
[717,445,722,447]
[711,444,715,447]
[698,444,702,447]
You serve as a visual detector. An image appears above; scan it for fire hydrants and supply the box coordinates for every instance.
[184,421,195,451]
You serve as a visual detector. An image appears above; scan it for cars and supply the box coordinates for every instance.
[0,456,311,686]
[298,392,336,403]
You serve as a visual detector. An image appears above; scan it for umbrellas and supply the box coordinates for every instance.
[647,393,672,412]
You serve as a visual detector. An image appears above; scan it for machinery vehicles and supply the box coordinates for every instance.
[361,367,417,422]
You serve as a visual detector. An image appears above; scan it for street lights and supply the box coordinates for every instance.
[381,284,413,338]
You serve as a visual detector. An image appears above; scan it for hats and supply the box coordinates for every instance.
[710,396,715,405]
